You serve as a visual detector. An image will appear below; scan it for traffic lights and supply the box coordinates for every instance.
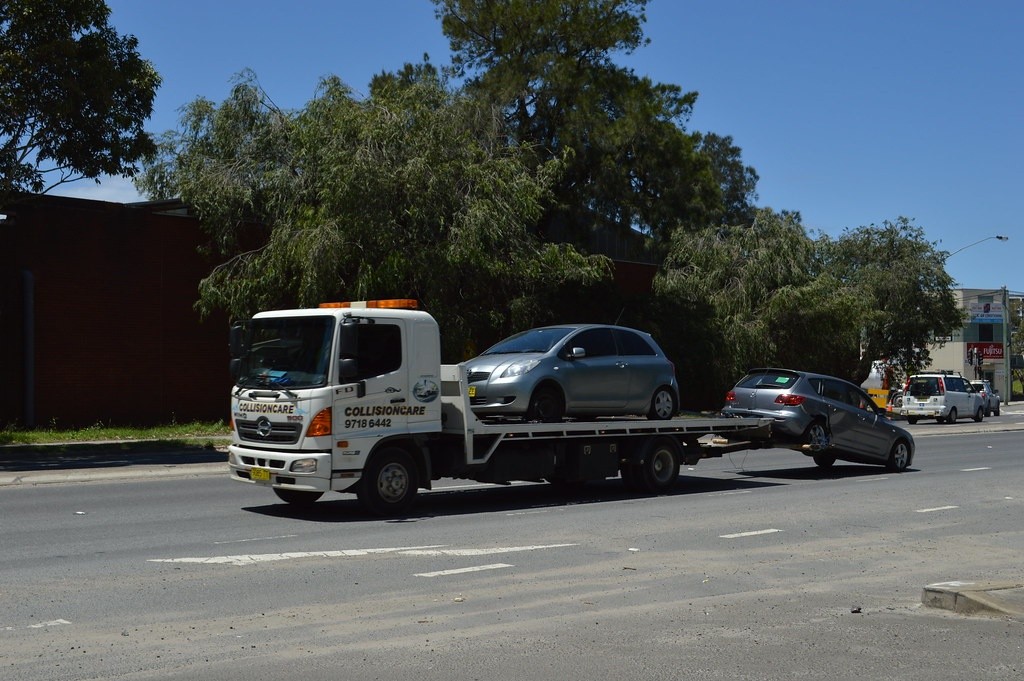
[978,352,983,365]
[967,350,973,366]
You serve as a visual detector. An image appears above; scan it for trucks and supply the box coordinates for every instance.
[226,300,825,515]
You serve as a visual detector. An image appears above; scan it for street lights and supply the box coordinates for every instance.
[939,236,1008,264]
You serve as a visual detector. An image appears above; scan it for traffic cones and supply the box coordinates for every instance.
[886,399,893,412]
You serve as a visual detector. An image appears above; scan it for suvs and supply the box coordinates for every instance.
[899,369,985,424]
[964,380,1001,417]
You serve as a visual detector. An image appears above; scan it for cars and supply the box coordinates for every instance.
[887,381,905,407]
[457,324,680,424]
[719,367,915,473]
